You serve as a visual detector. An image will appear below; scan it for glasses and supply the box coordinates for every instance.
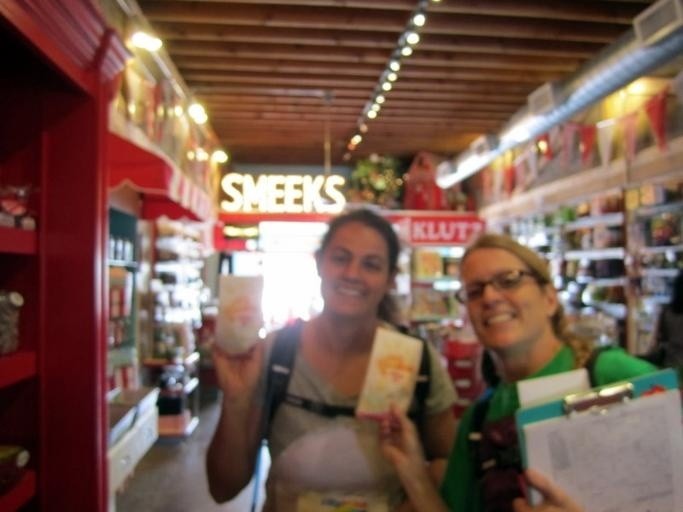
[455,269,534,305]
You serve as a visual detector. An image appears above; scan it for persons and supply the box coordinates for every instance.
[371,230,659,511]
[203,205,460,510]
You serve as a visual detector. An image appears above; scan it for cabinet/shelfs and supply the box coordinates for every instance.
[408,137,682,425]
[0,0,205,511]
